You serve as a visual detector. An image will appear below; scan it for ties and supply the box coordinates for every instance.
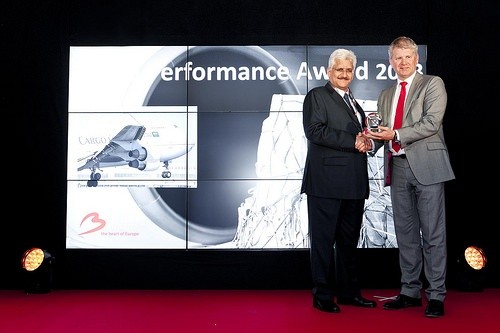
[392,81,408,153]
[344,92,355,115]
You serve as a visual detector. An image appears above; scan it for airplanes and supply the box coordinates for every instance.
[73,124,196,186]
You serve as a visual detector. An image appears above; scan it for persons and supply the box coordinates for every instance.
[358,36,457,318]
[300,48,377,314]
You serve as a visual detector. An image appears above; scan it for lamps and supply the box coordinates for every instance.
[22,246,61,292]
[465,247,500,290]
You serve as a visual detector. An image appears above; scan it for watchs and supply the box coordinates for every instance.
[393,132,397,142]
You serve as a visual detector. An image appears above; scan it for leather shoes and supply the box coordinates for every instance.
[425,299,444,318]
[383,294,422,310]
[336,289,377,307]
[313,298,340,312]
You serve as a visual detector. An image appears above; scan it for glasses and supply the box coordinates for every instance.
[329,67,352,72]
